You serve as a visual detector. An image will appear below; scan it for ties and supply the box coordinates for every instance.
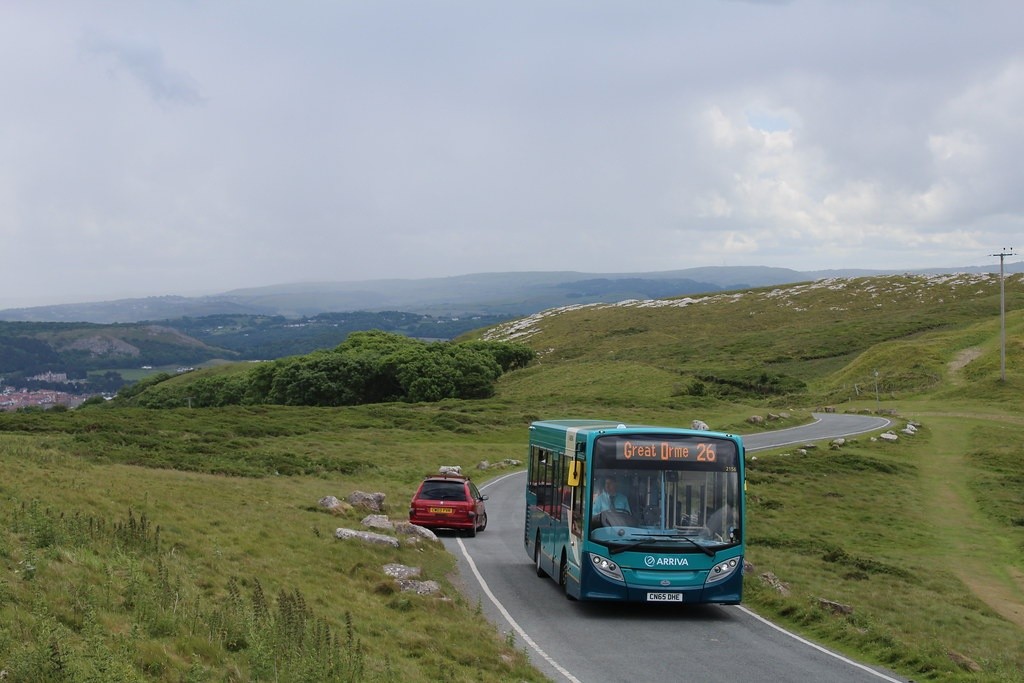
[610,497,616,511]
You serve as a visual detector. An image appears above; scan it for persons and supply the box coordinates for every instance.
[592,476,631,515]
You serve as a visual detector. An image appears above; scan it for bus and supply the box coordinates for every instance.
[523,419,747,606]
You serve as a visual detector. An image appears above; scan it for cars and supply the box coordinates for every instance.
[408,465,490,537]
